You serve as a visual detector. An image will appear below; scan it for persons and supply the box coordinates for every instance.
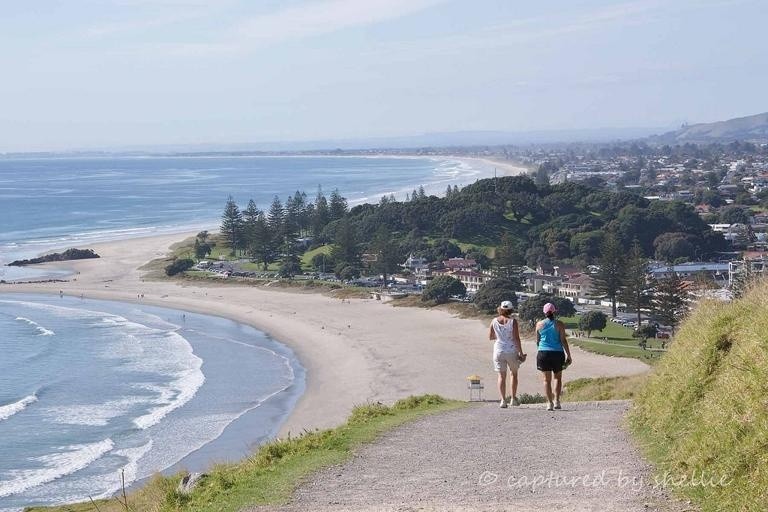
[488,301,526,408]
[536,303,572,411]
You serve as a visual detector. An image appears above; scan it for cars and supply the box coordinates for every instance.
[304,273,426,291]
[197,261,279,279]
[613,306,670,338]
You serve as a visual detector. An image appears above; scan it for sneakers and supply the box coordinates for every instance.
[499,400,507,407]
[553,400,562,409]
[546,403,553,410]
[510,398,521,406]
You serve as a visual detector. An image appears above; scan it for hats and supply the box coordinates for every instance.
[500,300,512,309]
[543,303,555,313]
